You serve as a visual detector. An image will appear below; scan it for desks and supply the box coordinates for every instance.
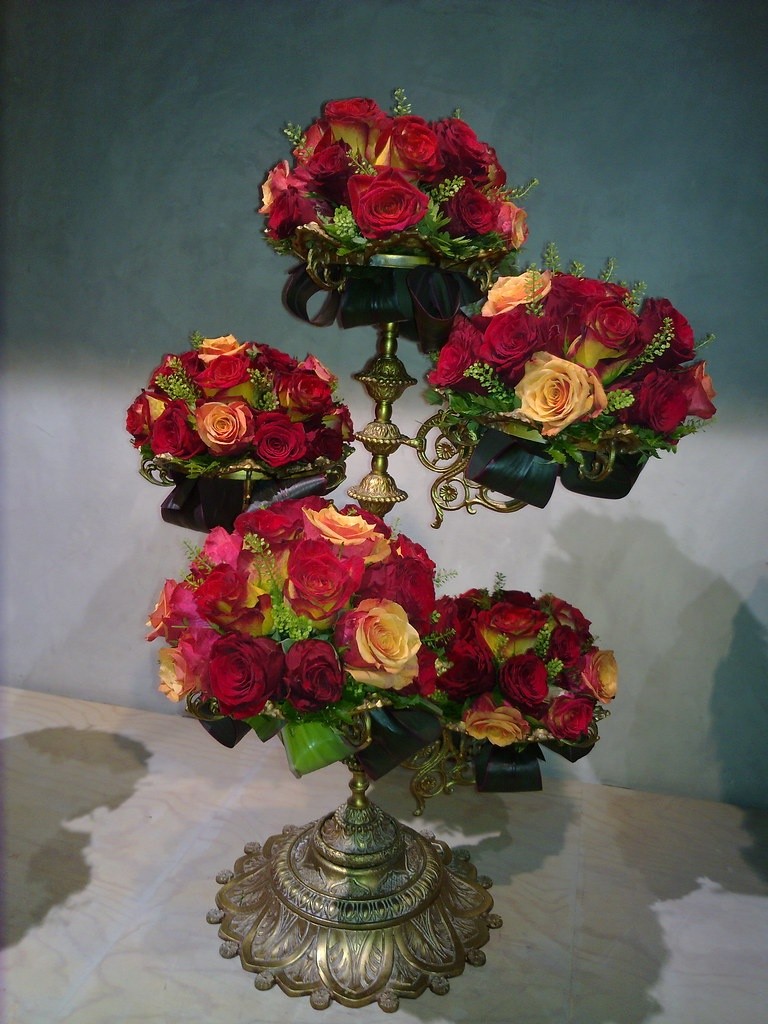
[0,684,762,1022]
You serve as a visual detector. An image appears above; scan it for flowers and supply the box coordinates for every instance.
[424,250,716,465]
[145,491,440,719]
[126,320,357,483]
[254,97,531,264]
[372,581,620,748]
